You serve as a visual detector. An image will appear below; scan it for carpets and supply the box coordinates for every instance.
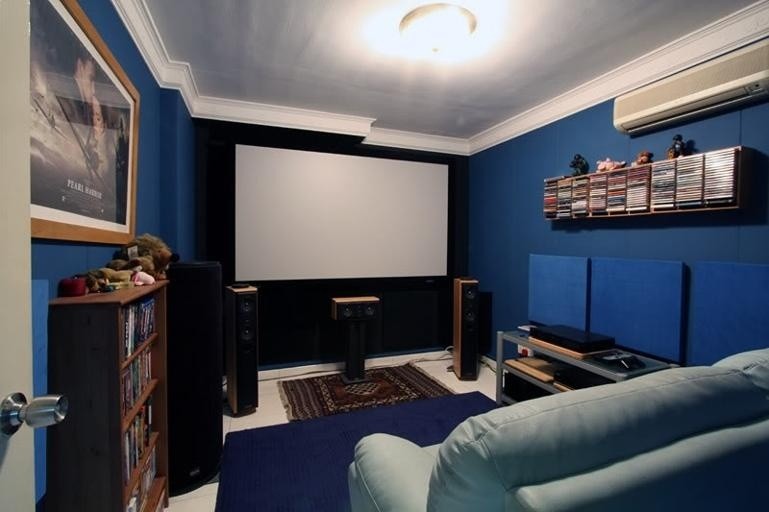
[276,359,456,422]
[213,388,503,512]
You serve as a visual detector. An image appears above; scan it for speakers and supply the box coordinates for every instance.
[333,296,383,322]
[227,285,260,416]
[452,276,479,380]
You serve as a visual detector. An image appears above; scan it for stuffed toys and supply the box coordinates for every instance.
[76,233,180,292]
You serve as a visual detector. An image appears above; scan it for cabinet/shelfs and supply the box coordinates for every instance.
[492,331,672,405]
[543,145,744,220]
[47,275,171,512]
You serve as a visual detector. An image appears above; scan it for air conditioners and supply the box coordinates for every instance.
[613,38,769,137]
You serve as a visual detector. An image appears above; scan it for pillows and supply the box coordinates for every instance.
[354,431,437,512]
[711,348,769,391]
[426,365,769,512]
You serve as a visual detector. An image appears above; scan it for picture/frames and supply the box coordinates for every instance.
[28,0,141,245]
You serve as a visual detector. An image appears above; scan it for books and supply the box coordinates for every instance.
[543,148,738,219]
[121,299,161,512]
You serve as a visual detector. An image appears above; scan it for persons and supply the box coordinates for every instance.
[48,42,118,175]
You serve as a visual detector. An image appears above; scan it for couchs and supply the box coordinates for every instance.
[349,347,769,512]
